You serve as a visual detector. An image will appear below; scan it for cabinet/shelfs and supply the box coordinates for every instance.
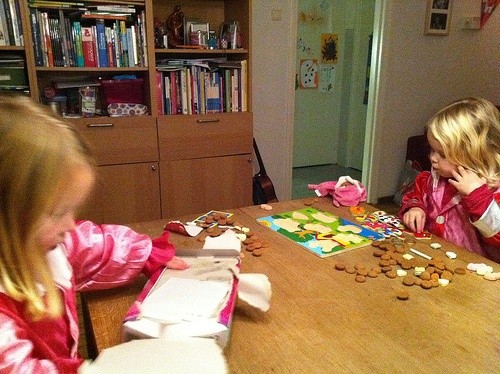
[0,0,251,119]
[157,112,253,220]
[67,118,161,227]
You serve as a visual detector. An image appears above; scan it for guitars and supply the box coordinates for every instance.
[253,138,279,205]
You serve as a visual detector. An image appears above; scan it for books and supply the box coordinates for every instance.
[157,57,249,115]
[0,0,149,69]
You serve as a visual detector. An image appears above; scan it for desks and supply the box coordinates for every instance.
[80,195,500,374]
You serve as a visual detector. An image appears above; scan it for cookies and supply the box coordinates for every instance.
[304,197,320,205]
[260,203,272,210]
[196,213,269,257]
[335,237,500,300]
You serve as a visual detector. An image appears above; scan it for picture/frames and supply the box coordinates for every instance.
[424,0,454,35]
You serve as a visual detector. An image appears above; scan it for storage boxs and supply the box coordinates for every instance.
[102,78,144,104]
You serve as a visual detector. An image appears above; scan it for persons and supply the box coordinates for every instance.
[398,96,500,264]
[0,94,189,374]
